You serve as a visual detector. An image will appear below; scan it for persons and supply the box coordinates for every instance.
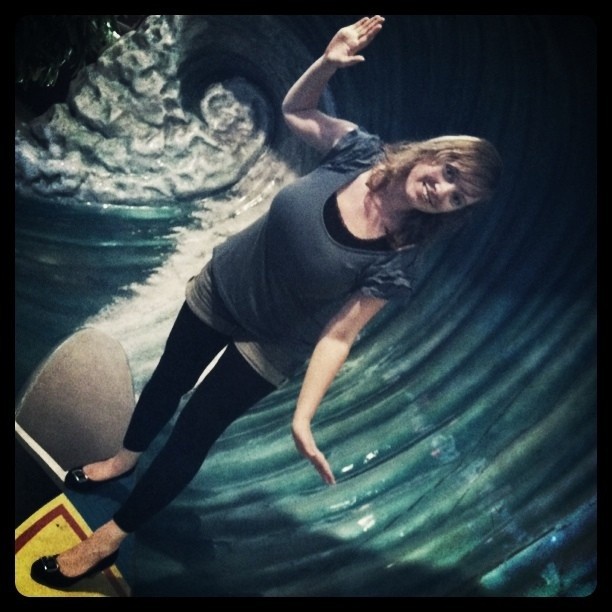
[30,14,501,590]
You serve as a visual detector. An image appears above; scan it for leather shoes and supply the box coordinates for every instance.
[31,547,119,588]
[65,457,136,490]
